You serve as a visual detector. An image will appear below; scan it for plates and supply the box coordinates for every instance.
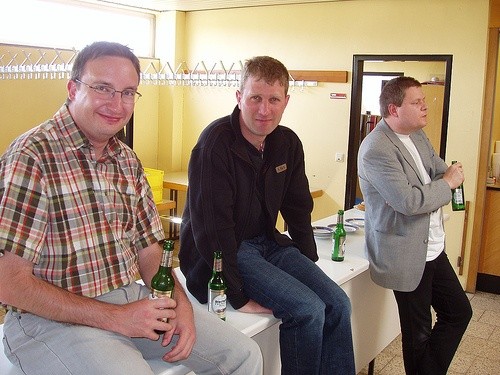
[311,226,334,238]
[328,223,358,232]
[345,218,365,226]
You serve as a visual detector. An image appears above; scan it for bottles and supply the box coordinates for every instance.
[331,210,347,261]
[209,251,226,322]
[450,161,467,211]
[148,239,175,334]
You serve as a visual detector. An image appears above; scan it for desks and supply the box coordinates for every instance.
[163,173,323,240]
[1,200,401,375]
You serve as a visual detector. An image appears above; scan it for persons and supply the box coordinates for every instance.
[355,76,473,375]
[0,42,264,375]
[178,55,354,375]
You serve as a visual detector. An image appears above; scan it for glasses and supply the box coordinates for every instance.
[72,77,142,104]
